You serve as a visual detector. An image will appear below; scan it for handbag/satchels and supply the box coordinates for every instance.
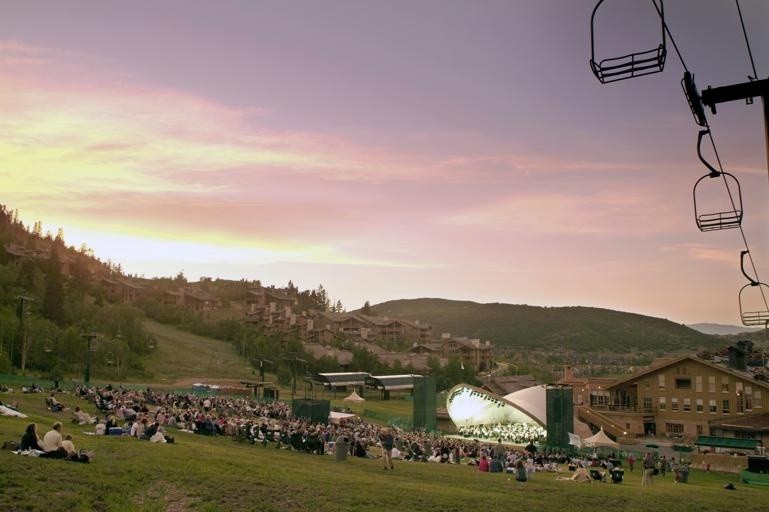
[2,440,20,453]
[653,467,658,476]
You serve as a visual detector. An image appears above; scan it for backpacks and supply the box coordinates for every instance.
[386,435,392,445]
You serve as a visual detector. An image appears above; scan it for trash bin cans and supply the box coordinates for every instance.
[335,435,351,460]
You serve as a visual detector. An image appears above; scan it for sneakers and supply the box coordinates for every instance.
[382,465,394,471]
